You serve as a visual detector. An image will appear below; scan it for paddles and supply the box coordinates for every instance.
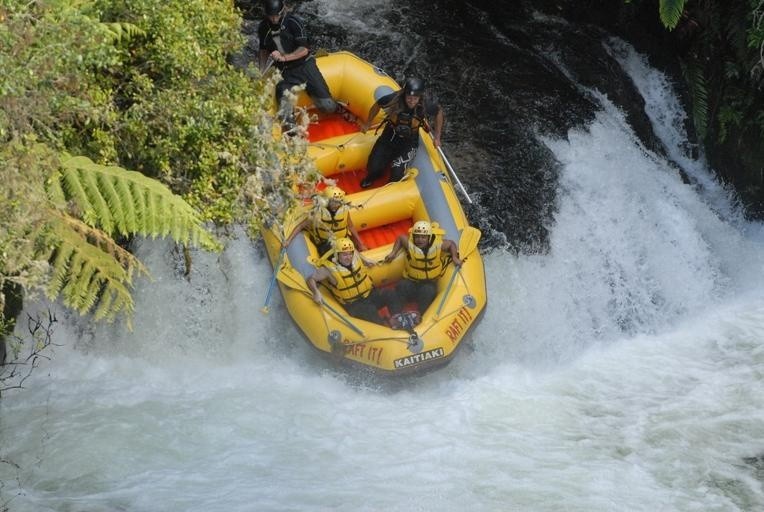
[259,207,301,313]
[275,268,368,340]
[431,227,481,320]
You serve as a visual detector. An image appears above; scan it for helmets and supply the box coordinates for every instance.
[322,185,344,209]
[411,220,433,245]
[403,76,425,97]
[264,0,286,17]
[334,237,355,262]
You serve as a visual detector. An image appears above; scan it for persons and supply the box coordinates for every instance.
[361,77,445,189]
[308,238,400,323]
[257,1,338,138]
[282,186,367,260]
[386,220,461,318]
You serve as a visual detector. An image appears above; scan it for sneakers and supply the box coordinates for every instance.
[360,175,374,187]
[336,104,354,122]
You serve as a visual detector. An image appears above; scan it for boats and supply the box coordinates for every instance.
[244,50,488,381]
